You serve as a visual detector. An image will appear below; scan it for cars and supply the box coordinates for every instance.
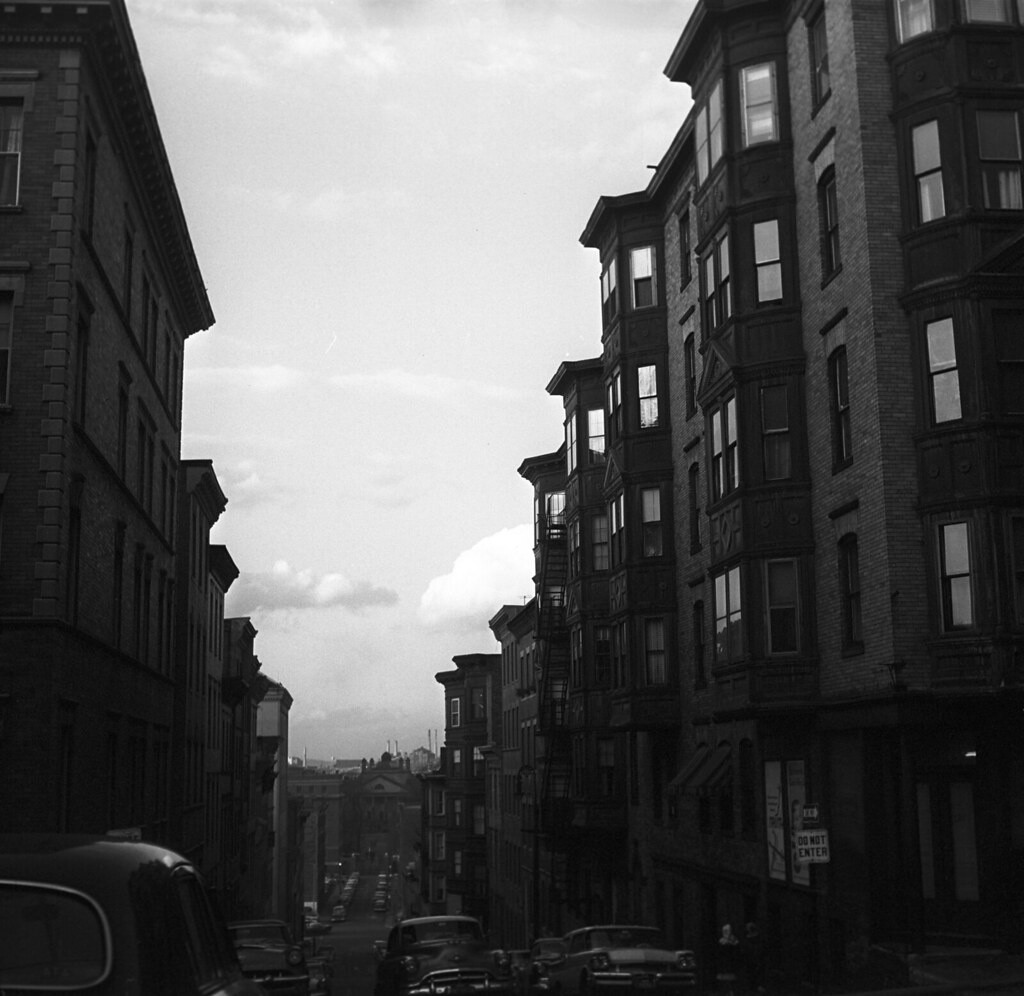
[227,920,307,996]
[0,824,269,996]
[330,871,396,925]
[305,959,334,995]
[374,913,696,996]
